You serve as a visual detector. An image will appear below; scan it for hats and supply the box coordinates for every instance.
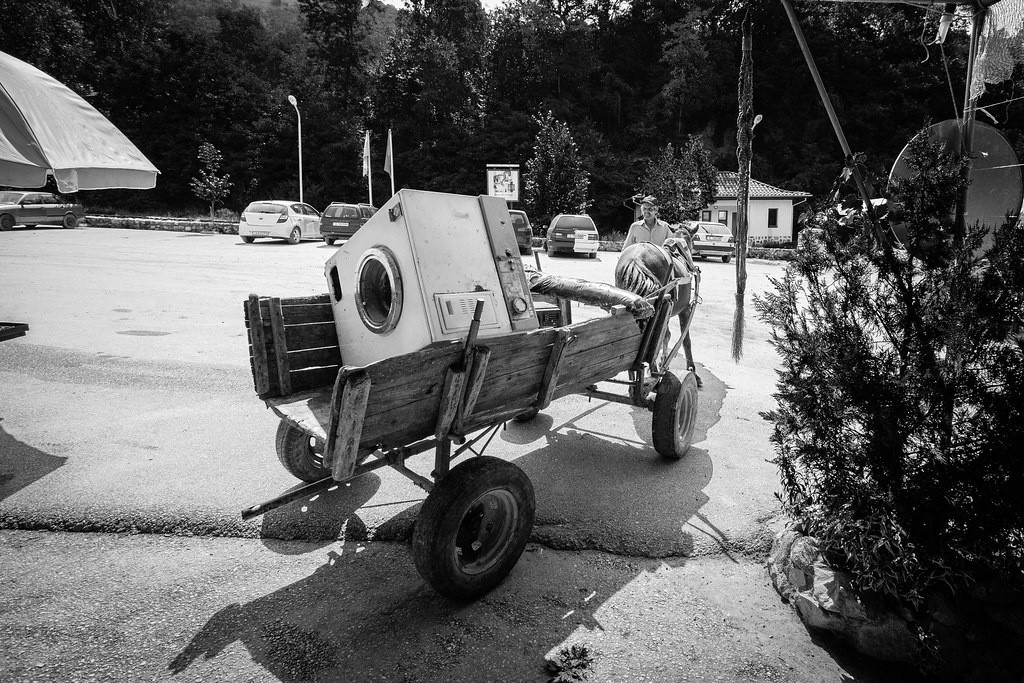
[640,195,659,207]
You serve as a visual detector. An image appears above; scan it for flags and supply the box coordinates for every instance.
[384,132,393,176]
[363,134,370,176]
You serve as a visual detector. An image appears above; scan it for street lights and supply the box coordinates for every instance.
[288,94,303,202]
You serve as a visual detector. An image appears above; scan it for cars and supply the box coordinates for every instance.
[680,221,735,263]
[0,191,85,231]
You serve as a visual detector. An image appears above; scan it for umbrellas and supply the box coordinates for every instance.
[0,51,162,194]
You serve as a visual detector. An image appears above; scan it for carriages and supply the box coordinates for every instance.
[243,224,703,603]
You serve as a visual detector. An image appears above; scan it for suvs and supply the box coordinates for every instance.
[319,201,380,246]
[508,209,534,255]
[542,213,599,259]
[238,200,324,245]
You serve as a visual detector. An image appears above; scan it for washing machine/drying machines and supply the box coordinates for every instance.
[322,187,539,375]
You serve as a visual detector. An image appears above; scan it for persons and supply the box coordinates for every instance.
[623,196,674,253]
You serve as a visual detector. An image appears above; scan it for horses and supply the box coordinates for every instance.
[615,224,699,374]
